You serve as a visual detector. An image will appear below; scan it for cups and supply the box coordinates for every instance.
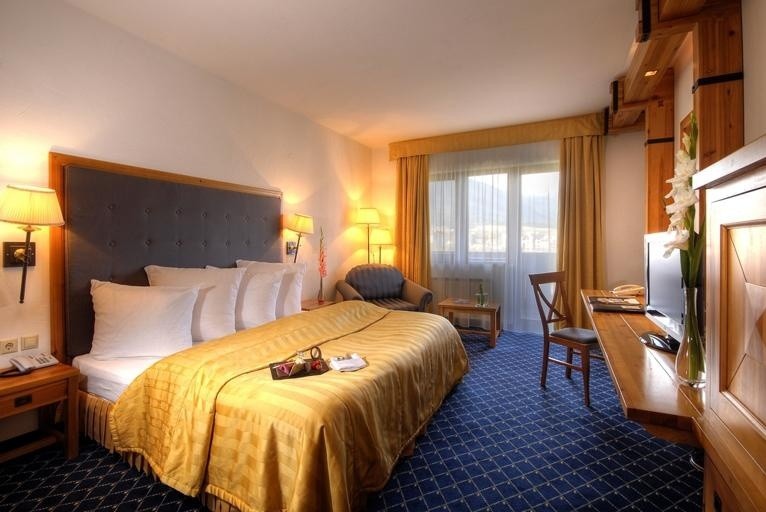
[475,293,482,308]
[481,293,488,308]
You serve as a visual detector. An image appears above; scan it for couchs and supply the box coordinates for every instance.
[338,264,434,314]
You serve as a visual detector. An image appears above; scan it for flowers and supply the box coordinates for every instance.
[668,116,710,389]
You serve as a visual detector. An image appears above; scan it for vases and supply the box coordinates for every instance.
[674,286,709,388]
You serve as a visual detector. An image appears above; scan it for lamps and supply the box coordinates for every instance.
[0,182,65,304]
[288,213,315,263]
[372,229,393,265]
[355,207,380,268]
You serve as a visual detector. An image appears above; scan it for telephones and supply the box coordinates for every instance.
[0,350,60,377]
[613,284,645,295]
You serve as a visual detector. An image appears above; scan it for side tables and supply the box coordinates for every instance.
[437,294,503,348]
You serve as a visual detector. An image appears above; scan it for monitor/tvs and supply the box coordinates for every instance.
[643,230,686,343]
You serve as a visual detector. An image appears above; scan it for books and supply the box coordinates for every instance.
[588,296,645,313]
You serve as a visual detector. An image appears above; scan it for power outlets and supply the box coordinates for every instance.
[22,335,39,350]
[0,338,18,354]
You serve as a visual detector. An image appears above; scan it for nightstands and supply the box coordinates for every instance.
[0,354,81,462]
[301,295,331,309]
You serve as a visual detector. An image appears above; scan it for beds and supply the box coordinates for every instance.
[70,260,457,512]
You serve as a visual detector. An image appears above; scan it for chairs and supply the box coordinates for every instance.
[526,272,603,405]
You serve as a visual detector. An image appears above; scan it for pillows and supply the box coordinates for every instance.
[262,258,303,318]
[222,264,282,331]
[86,278,202,362]
[140,267,242,341]
